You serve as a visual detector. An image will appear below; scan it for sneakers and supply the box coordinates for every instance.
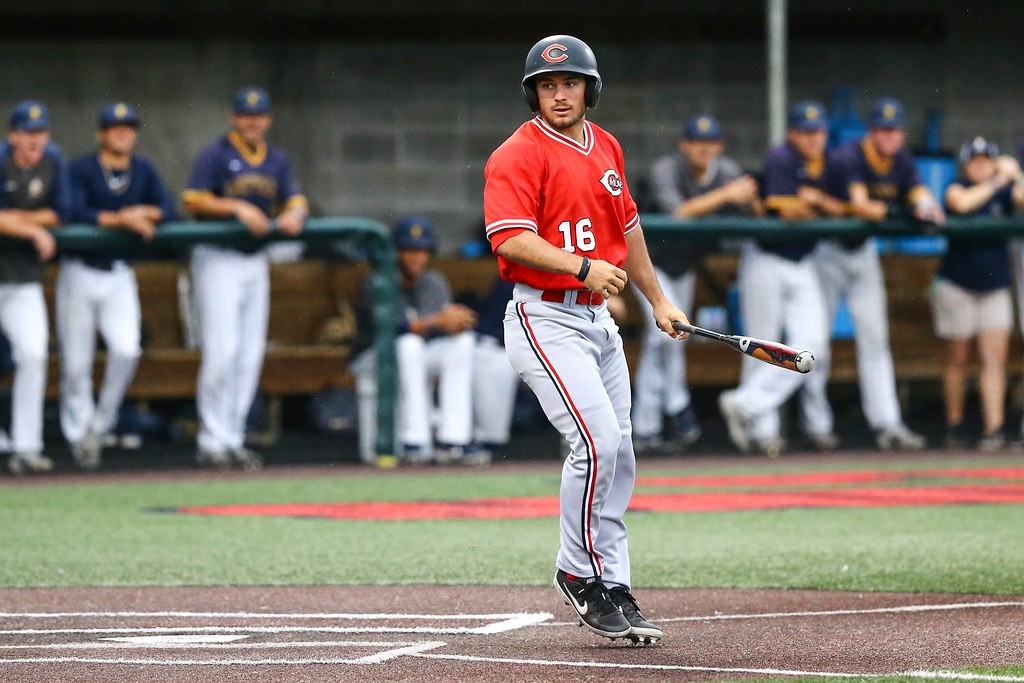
[554,569,631,640]
[607,587,664,644]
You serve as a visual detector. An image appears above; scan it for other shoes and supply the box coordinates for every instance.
[634,406,1007,453]
[2,437,261,475]
[401,439,503,468]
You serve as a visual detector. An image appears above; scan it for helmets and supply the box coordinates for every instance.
[522,35,602,114]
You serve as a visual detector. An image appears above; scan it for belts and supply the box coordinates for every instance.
[542,288,604,306]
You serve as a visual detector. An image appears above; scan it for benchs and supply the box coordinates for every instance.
[0,254,1023,447]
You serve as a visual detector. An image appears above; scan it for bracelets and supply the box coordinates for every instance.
[575,255,588,279]
[579,259,591,282]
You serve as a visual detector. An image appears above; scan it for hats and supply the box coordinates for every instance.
[232,87,271,118]
[958,134,999,167]
[683,115,724,143]
[8,100,54,132]
[98,100,139,131]
[395,218,438,251]
[870,98,908,132]
[789,102,829,132]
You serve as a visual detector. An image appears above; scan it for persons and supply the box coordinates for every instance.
[55,103,179,469]
[926,133,1024,452]
[629,112,762,454]
[474,271,627,462]
[345,217,475,468]
[719,101,852,455]
[179,86,309,472]
[484,34,693,644]
[800,100,926,451]
[0,100,74,476]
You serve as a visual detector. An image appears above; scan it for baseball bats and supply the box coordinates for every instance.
[655,319,816,374]
